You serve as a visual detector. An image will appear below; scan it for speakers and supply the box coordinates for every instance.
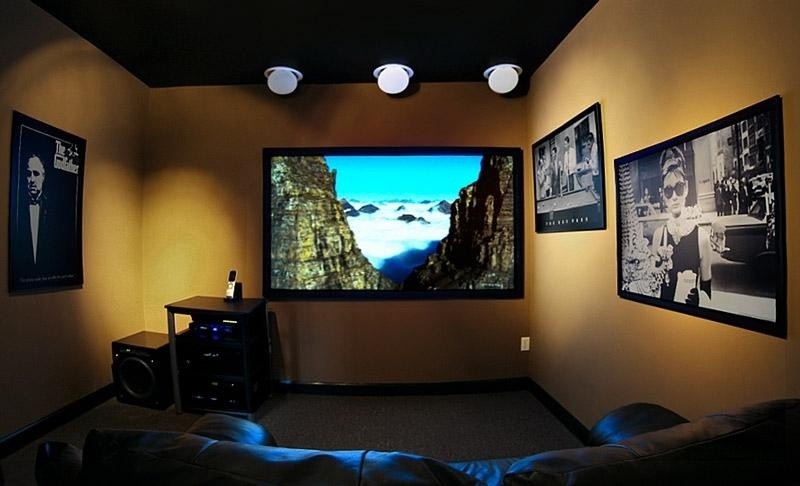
[110,329,175,411]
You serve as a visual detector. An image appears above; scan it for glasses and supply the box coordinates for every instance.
[661,179,687,200]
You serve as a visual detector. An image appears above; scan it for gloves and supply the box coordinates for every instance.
[685,278,713,306]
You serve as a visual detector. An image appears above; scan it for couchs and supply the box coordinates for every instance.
[185,403,691,486]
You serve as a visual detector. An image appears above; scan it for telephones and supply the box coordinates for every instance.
[223,270,242,305]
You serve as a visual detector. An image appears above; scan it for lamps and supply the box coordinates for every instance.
[483,63,523,95]
[264,66,303,94]
[373,64,414,94]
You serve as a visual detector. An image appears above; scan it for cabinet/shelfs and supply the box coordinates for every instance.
[164,296,267,423]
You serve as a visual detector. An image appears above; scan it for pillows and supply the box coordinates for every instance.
[34,440,82,485]
[503,399,800,486]
[82,428,486,486]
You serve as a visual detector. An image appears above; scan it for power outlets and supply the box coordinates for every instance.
[521,336,530,352]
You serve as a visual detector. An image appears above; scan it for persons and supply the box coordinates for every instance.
[19,154,49,279]
[650,147,713,307]
[536,159,548,199]
[582,133,598,193]
[641,188,650,206]
[547,147,563,195]
[714,175,752,216]
[563,136,577,190]
[656,186,664,215]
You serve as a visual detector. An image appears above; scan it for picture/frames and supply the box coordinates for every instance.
[530,97,611,232]
[616,92,792,345]
[8,108,84,295]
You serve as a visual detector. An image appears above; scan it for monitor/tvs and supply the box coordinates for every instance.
[260,142,526,304]
